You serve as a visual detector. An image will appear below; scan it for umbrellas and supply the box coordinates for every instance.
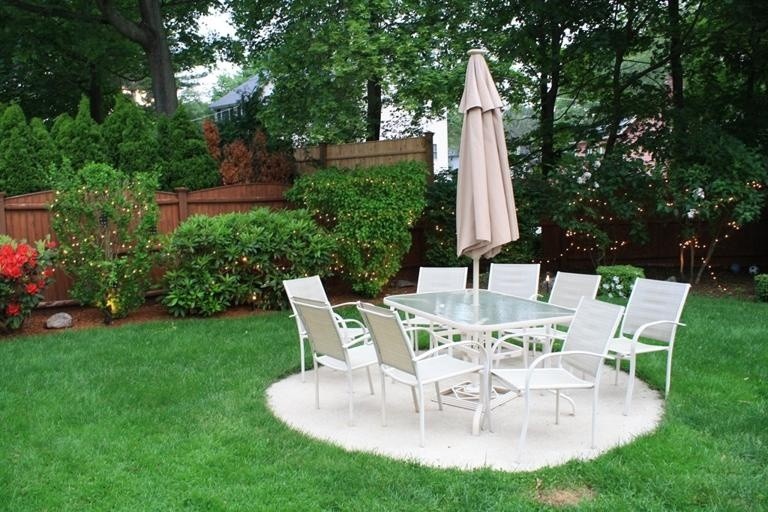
[455,49,520,302]
